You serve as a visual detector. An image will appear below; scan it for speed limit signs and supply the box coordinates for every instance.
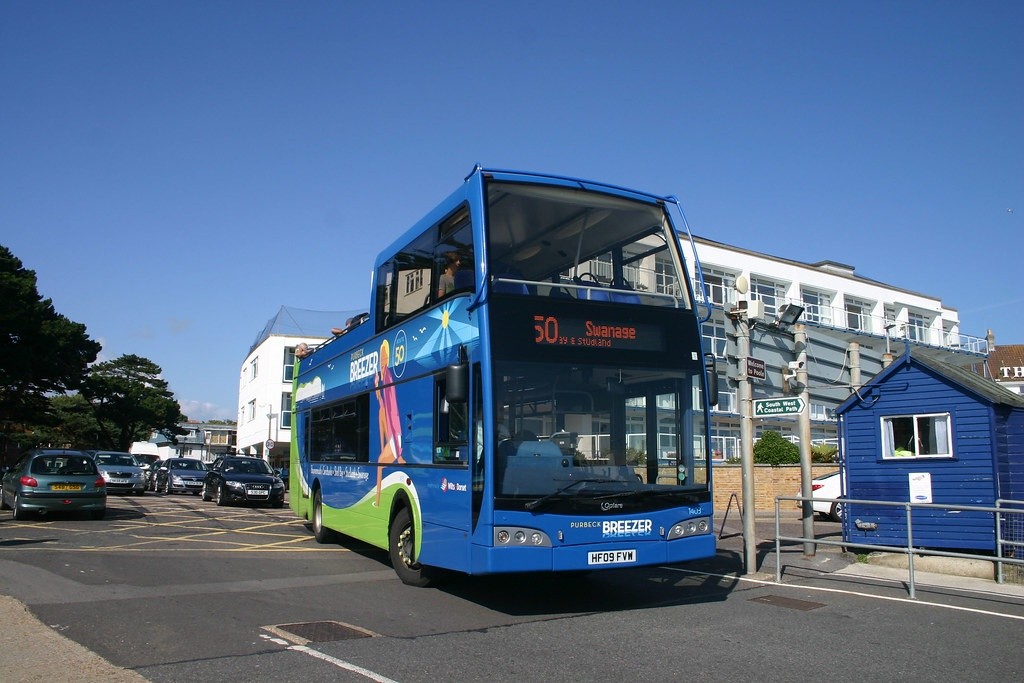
[266,438,276,450]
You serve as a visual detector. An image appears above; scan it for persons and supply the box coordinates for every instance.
[331,318,352,337]
[295,343,313,359]
[460,400,511,463]
[437,250,463,298]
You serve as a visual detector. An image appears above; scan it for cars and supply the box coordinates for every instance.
[92,450,144,495]
[797,469,849,524]
[155,457,208,495]
[144,460,168,493]
[0,448,107,520]
[202,456,285,508]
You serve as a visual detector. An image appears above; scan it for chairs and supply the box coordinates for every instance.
[318,268,642,495]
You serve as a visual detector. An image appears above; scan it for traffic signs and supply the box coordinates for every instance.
[746,356,767,380]
[753,396,806,418]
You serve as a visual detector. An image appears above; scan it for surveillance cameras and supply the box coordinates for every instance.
[784,361,804,380]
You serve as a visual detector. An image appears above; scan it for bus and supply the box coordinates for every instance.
[287,162,725,588]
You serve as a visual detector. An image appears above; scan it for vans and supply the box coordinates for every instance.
[132,453,162,491]
